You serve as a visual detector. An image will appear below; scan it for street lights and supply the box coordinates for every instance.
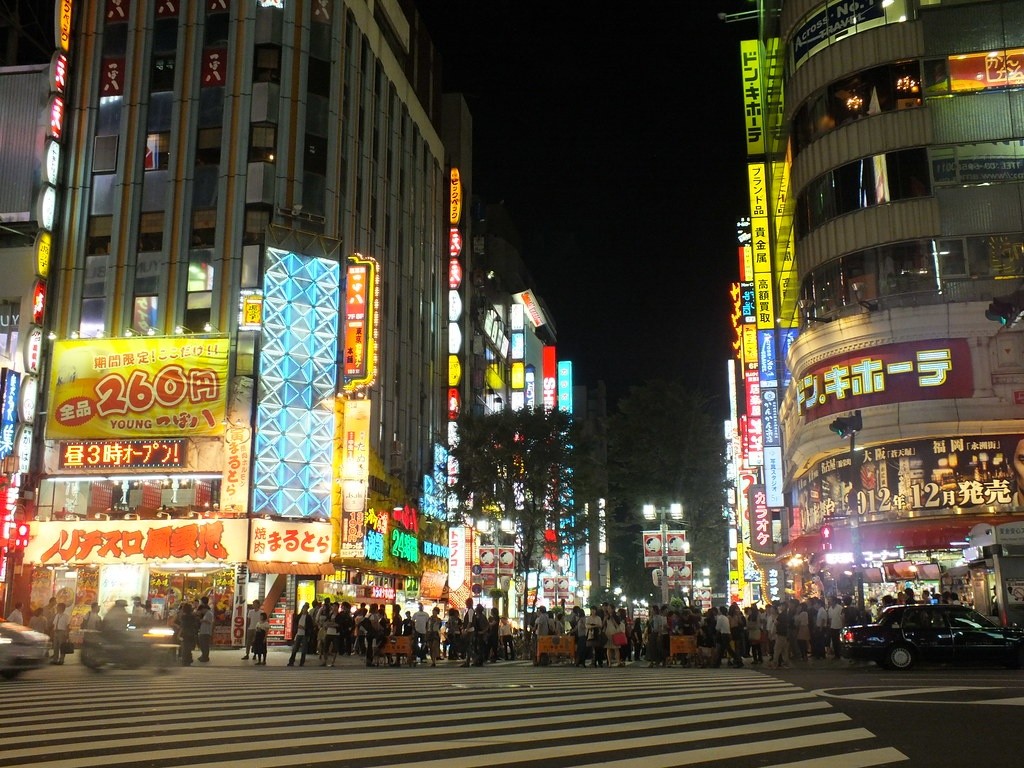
[541,557,567,607]
[643,503,684,605]
[475,511,518,610]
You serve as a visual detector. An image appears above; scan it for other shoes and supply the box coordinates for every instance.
[198,656,209,662]
[319,653,839,668]
[299,663,303,667]
[242,656,249,660]
[255,662,260,665]
[252,655,256,660]
[287,663,294,666]
[261,662,266,665]
[51,661,62,665]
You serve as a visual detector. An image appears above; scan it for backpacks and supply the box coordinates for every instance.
[357,614,376,634]
[631,629,637,641]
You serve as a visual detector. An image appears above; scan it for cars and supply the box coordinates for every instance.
[0,617,54,680]
[839,603,1024,672]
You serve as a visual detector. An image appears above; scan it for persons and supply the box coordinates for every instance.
[1004,434,1024,507]
[131,597,160,627]
[251,612,271,665]
[83,600,128,631]
[29,608,48,633]
[531,588,960,668]
[51,603,70,665]
[7,601,24,625]
[175,596,213,666]
[43,597,57,636]
[241,600,264,660]
[287,597,515,668]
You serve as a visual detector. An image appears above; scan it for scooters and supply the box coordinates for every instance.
[81,625,154,670]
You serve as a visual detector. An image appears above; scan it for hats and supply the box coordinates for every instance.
[991,586,996,590]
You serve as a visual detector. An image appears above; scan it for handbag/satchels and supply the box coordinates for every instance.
[317,627,327,641]
[421,641,428,652]
[612,629,627,645]
[60,642,74,654]
[548,628,553,635]
[748,627,761,640]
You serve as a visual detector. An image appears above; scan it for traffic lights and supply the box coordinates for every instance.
[820,524,834,553]
[829,414,860,441]
